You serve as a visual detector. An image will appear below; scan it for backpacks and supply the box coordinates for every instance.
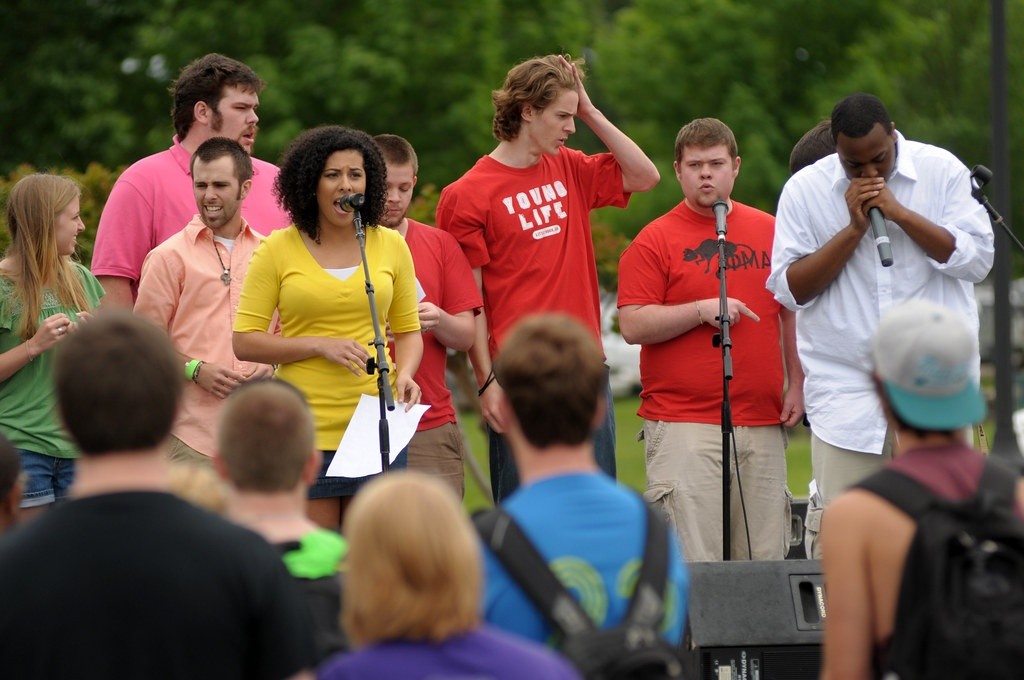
[468,491,688,680]
[849,453,1023,680]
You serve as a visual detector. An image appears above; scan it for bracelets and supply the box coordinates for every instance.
[193,362,206,384]
[478,370,493,393]
[184,358,202,380]
[26,339,33,362]
[478,376,495,396]
[695,300,704,325]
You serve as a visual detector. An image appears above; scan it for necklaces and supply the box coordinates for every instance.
[211,236,231,286]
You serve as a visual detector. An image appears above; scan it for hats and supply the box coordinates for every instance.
[871,299,988,429]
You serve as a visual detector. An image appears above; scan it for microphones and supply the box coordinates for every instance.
[712,200,730,242]
[340,193,364,212]
[868,206,894,266]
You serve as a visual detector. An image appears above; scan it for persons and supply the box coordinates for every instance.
[372,133,484,500]
[0,172,106,522]
[0,311,317,680]
[470,308,689,654]
[133,136,282,474]
[232,124,424,532]
[206,373,348,625]
[91,52,292,323]
[436,52,661,508]
[818,300,1024,680]
[764,93,994,564]
[618,118,806,563]
[288,468,583,680]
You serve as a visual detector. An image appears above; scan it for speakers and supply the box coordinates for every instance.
[683,560,827,680]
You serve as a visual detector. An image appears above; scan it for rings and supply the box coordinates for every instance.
[57,327,62,335]
